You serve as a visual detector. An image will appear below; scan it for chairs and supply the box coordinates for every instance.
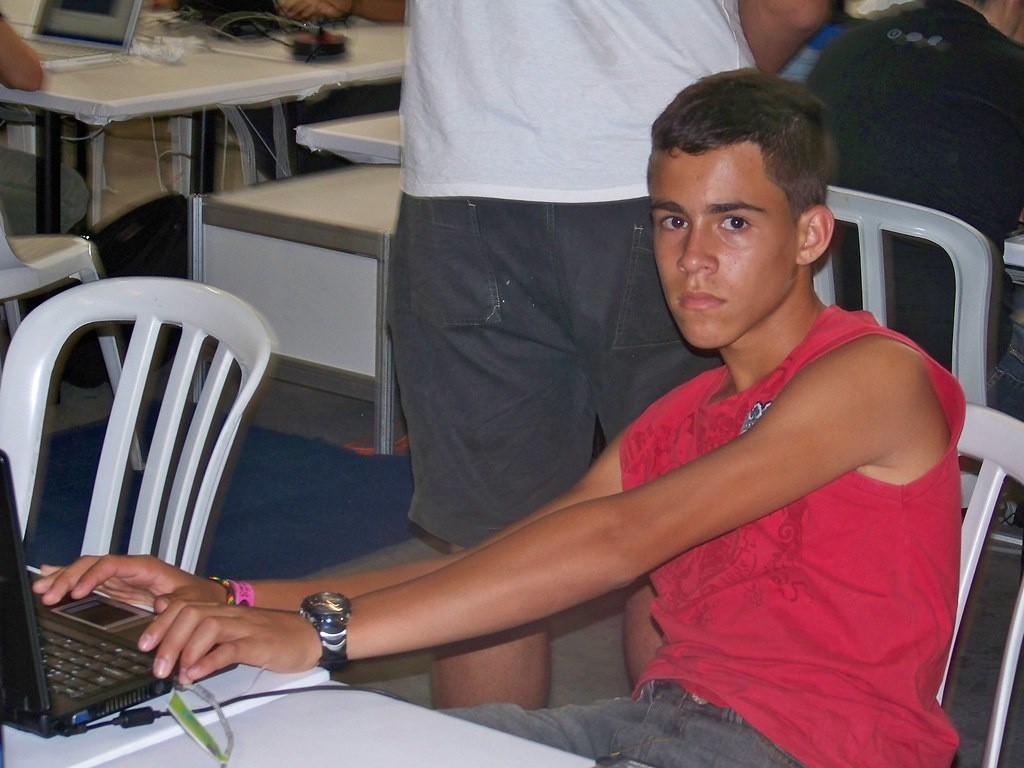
[0,257,272,588]
[812,186,993,508]
[936,402,1024,768]
[0,210,145,471]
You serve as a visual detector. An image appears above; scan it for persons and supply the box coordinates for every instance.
[0,13,90,236]
[801,0,1024,529]
[154,0,406,181]
[386,0,832,711]
[31,67,966,767]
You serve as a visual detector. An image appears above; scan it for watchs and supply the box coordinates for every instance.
[300,590,353,665]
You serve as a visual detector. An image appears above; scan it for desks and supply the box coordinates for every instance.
[1,0,417,456]
[0,659,597,768]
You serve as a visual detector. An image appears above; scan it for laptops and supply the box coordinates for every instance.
[21,0,142,69]
[0,450,238,734]
[175,0,349,38]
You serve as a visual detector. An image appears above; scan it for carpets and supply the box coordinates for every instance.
[22,395,423,580]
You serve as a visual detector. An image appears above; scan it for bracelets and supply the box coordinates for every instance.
[209,576,255,607]
